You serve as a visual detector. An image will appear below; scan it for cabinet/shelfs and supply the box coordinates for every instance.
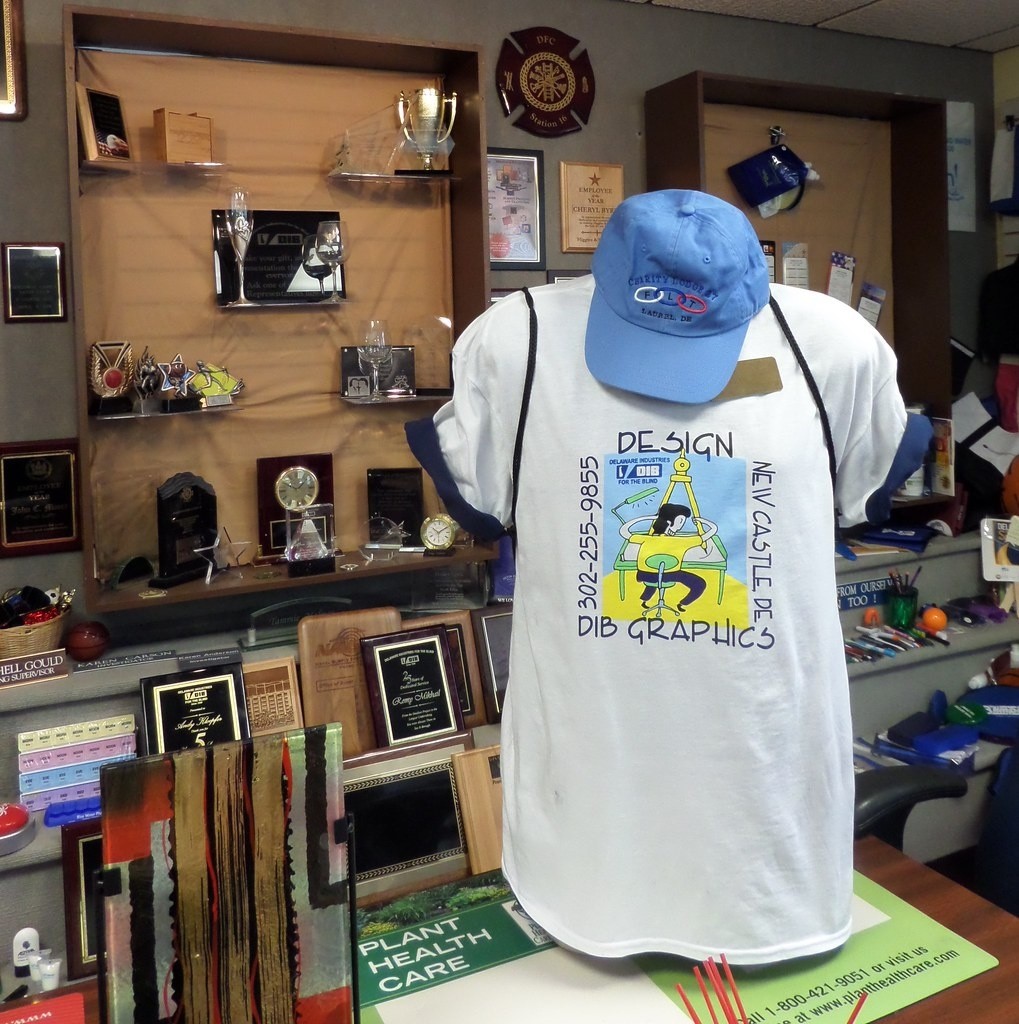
[0,531,1019,1003]
[60,2,503,618]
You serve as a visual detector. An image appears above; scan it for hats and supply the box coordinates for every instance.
[585,188,771,407]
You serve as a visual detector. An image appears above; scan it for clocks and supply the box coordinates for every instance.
[419,514,459,557]
[253,453,343,565]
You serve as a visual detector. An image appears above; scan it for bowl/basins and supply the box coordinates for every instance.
[67,621,109,663]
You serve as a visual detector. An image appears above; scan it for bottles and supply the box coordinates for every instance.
[38,959,62,993]
[356,320,393,402]
[13,927,39,978]
[27,949,52,985]
[897,465,924,496]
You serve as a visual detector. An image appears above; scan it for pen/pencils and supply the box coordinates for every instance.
[897,574,903,589]
[889,572,900,593]
[844,623,951,663]
[909,566,922,587]
[905,574,908,587]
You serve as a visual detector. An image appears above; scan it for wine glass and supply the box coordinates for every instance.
[315,221,352,304]
[302,236,333,303]
[227,189,257,306]
[358,354,392,397]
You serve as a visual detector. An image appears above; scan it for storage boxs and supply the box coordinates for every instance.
[153,108,212,166]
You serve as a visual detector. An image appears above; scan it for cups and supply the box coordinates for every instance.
[886,586,918,630]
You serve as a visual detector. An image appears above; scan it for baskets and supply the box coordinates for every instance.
[0,585,72,659]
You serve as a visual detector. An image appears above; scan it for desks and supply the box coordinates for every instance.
[0,836,1019,1024]
